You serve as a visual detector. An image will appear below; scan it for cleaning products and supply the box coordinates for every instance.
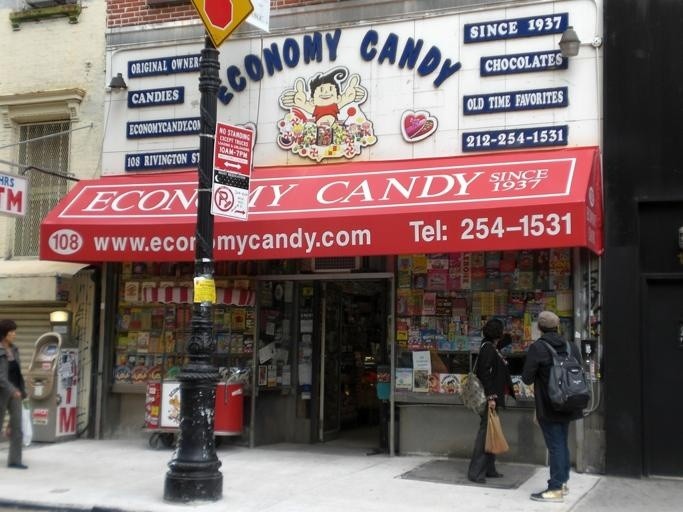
[144,377,249,448]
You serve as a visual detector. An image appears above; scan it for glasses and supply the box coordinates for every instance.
[559,25,601,57]
[108,73,128,91]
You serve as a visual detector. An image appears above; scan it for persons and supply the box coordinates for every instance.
[278,64,367,122]
[521,310,581,504]
[0,316,31,471]
[466,319,515,485]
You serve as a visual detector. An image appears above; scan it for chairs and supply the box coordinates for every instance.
[8,462,28,469]
[531,481,569,502]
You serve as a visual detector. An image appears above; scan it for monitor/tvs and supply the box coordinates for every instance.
[111,299,254,387]
[394,246,574,396]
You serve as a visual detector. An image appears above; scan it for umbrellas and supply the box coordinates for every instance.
[476,475,486,484]
[487,470,502,478]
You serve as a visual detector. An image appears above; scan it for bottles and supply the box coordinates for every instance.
[539,311,559,328]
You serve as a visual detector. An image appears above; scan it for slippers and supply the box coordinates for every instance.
[461,342,491,416]
[541,337,590,410]
